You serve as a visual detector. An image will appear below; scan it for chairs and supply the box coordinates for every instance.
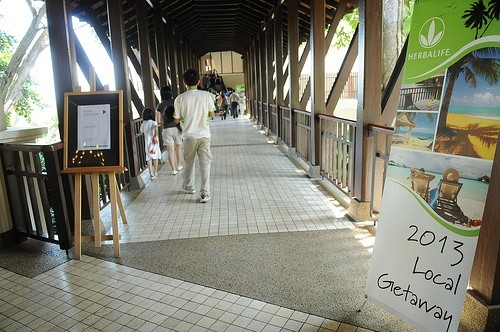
[410,169,463,218]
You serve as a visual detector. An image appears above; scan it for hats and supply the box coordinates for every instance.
[442,168,460,183]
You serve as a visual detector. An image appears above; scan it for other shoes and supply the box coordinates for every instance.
[150,176,155,179]
[154,173,158,176]
[177,165,183,171]
[172,171,178,175]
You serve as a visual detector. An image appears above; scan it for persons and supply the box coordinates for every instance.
[139,106,161,179]
[211,86,246,121]
[157,86,187,175]
[172,69,216,202]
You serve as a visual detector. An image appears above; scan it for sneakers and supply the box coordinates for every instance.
[199,194,211,203]
[182,185,196,194]
